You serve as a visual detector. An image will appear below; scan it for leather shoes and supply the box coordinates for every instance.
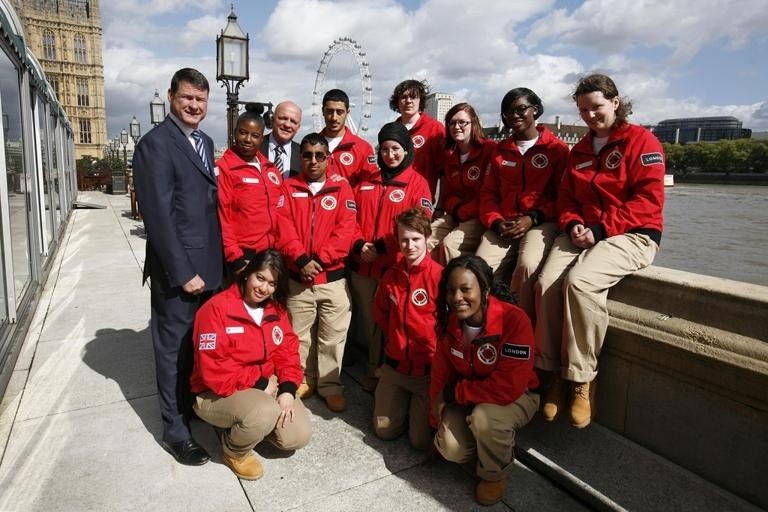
[162,439,209,465]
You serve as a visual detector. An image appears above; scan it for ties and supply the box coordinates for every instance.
[191,129,209,174]
[274,145,283,180]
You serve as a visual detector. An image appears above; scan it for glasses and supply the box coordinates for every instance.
[445,122,472,126]
[301,153,326,162]
[504,106,534,115]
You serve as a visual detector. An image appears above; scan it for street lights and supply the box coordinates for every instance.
[102,115,143,220]
[150,87,166,130]
[212,0,250,149]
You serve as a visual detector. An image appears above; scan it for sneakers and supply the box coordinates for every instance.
[297,384,314,398]
[327,395,346,411]
[569,382,594,429]
[222,454,263,479]
[543,378,563,421]
[476,478,508,505]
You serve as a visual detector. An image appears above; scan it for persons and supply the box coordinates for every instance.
[258,101,303,181]
[531,74,667,430]
[131,68,222,466]
[430,255,540,506]
[280,133,356,412]
[426,104,497,269]
[319,88,377,189]
[190,248,312,480]
[215,111,283,294]
[355,122,432,394]
[370,209,445,452]
[475,87,569,323]
[387,78,445,203]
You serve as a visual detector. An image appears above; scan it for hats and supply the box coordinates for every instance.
[378,123,410,152]
